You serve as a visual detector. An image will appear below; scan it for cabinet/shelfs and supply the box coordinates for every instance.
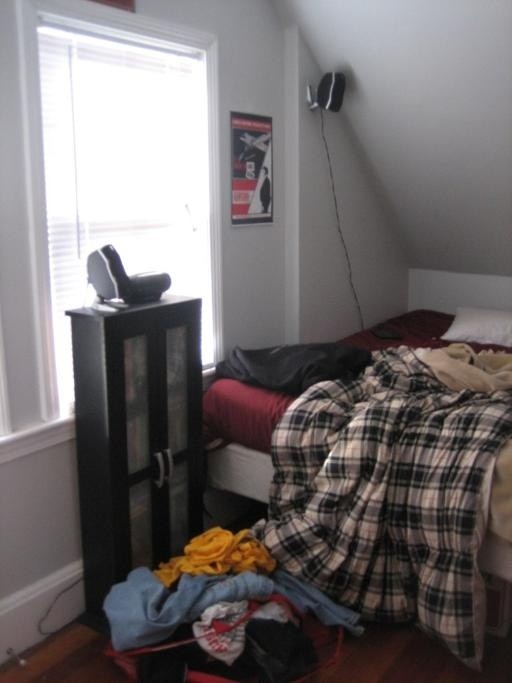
[64,291,204,638]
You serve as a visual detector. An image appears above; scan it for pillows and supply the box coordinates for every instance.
[441,306,512,350]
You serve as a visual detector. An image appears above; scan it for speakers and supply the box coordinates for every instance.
[86,244,132,300]
[316,71,346,113]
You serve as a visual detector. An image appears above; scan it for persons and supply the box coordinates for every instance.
[259,166,271,213]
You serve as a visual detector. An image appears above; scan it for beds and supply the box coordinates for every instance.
[208,270,512,587]
